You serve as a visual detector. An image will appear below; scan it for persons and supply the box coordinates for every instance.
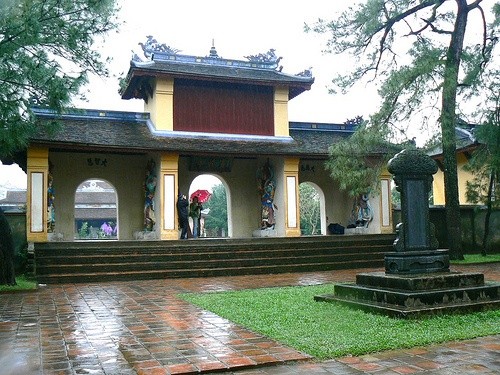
[177,188,190,239]
[189,196,200,238]
[197,203,203,236]
